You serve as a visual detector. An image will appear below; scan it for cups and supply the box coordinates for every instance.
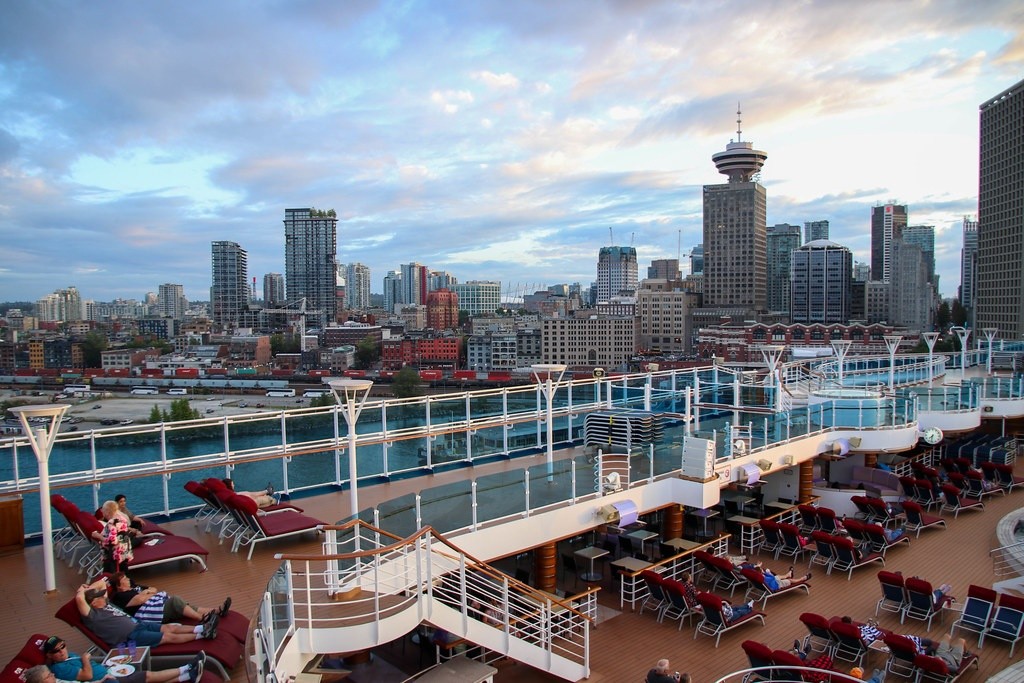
[117,643,126,655]
[128,641,136,658]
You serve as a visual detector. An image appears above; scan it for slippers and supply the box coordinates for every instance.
[803,573,812,581]
[789,566,793,578]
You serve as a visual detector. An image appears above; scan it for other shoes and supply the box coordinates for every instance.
[877,671,886,683]
[793,639,800,652]
[804,644,811,655]
[963,650,971,658]
[266,482,281,505]
[872,669,879,678]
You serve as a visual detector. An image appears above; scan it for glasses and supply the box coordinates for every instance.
[51,643,66,654]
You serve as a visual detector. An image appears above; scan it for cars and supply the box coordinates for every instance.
[0,403,134,433]
[204,396,265,414]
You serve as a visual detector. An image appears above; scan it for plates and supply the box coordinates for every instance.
[108,664,135,677]
[106,655,132,666]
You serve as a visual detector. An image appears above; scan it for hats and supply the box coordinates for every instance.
[85,588,107,604]
[850,667,864,679]
[44,636,65,652]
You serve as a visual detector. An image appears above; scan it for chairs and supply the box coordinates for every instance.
[0,476,331,683]
[388,454,1024,683]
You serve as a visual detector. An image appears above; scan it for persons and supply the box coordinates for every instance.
[107,572,233,626]
[44,635,207,683]
[648,502,972,681]
[114,495,146,530]
[223,479,281,507]
[91,500,157,596]
[24,664,119,683]
[75,580,220,650]
[436,595,521,656]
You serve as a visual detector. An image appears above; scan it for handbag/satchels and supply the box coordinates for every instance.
[101,540,120,574]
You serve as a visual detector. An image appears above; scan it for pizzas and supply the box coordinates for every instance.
[111,655,133,664]
[116,668,129,675]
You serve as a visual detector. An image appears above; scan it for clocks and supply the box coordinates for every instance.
[916,426,943,444]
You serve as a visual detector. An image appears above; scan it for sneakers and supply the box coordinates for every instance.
[187,649,207,671]
[188,660,203,683]
[198,596,231,639]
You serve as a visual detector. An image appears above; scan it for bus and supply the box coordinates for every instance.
[63,384,91,393]
[302,389,333,397]
[128,385,158,395]
[266,388,296,397]
[166,388,187,395]
